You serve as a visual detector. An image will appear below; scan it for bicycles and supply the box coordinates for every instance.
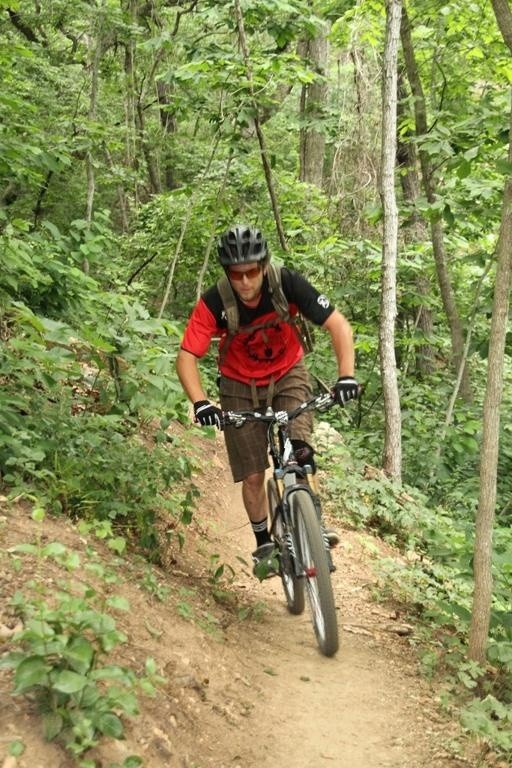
[193,384,366,657]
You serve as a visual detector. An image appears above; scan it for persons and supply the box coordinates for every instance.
[175,224,360,579]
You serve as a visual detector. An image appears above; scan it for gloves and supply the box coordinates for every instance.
[192,398,229,432]
[333,375,361,409]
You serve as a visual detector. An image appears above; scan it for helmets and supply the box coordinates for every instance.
[217,225,269,266]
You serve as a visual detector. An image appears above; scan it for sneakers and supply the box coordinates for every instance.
[323,523,340,547]
[250,542,277,579]
